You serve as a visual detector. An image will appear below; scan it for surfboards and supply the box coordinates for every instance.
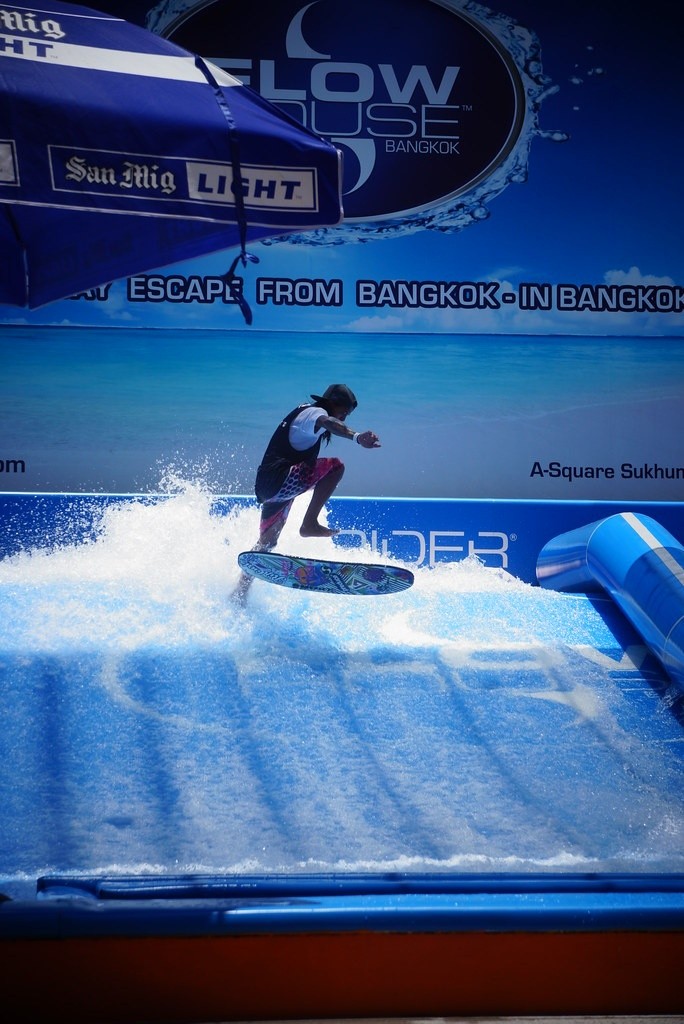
[236,550,416,597]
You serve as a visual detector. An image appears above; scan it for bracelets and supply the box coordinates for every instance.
[353,432,360,444]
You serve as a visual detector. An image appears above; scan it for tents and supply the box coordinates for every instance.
[0,0,344,325]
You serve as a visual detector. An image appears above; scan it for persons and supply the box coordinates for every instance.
[233,384,382,598]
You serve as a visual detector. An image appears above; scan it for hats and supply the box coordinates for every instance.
[310,384,358,409]
